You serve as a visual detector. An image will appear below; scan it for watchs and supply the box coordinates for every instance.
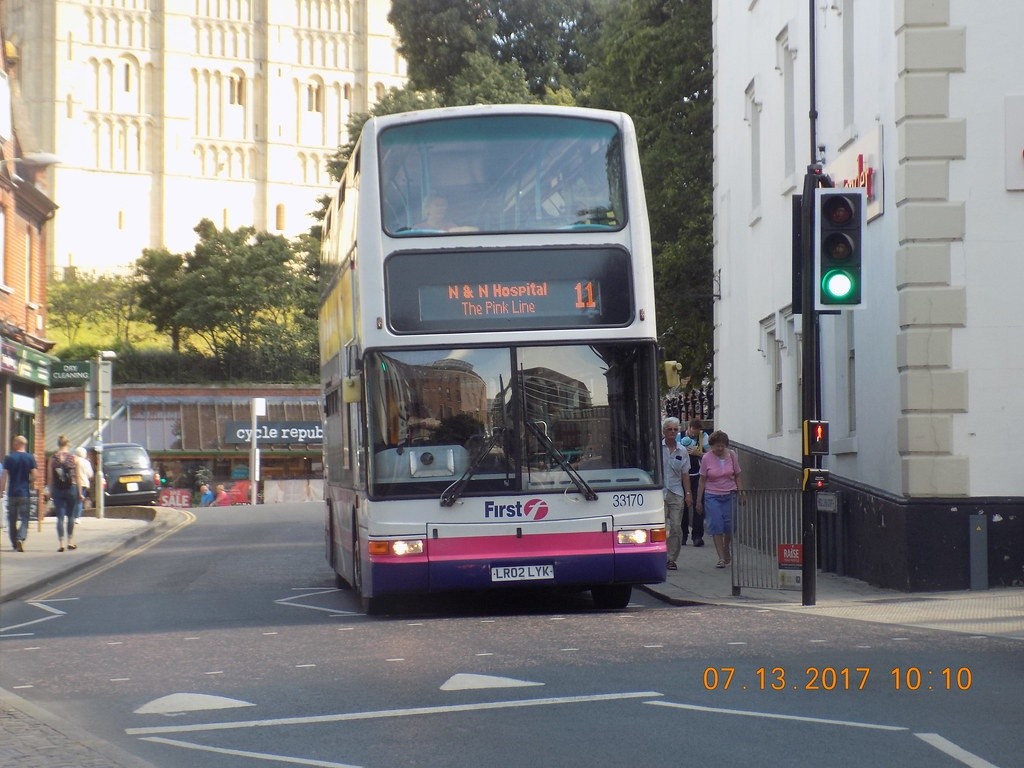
[685,491,691,494]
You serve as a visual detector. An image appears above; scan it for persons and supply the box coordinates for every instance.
[0,436,38,552]
[406,381,442,439]
[661,417,693,569]
[676,419,710,547]
[197,484,229,507]
[696,430,747,568]
[47,434,85,552]
[72,447,94,524]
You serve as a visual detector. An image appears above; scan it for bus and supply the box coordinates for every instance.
[321,103,671,615]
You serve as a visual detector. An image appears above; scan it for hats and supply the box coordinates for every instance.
[680,436,694,449]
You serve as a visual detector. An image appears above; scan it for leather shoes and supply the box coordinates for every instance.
[693,536,703,546]
[682,539,685,545]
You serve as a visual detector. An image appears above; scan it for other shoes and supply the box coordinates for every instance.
[58,548,64,552]
[67,545,76,549]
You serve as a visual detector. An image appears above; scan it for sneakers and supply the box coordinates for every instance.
[716,558,723,568]
[16,539,24,552]
[666,560,676,570]
[74,517,79,524]
[723,547,730,563]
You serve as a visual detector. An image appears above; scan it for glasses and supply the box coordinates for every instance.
[665,427,677,432]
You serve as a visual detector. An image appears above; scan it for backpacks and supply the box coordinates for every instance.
[50,452,74,490]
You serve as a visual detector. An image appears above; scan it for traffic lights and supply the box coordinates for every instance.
[792,186,866,316]
[804,421,829,456]
[160,470,169,490]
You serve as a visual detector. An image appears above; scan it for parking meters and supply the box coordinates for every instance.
[803,468,830,491]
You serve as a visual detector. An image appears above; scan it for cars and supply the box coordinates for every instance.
[84,441,160,510]
